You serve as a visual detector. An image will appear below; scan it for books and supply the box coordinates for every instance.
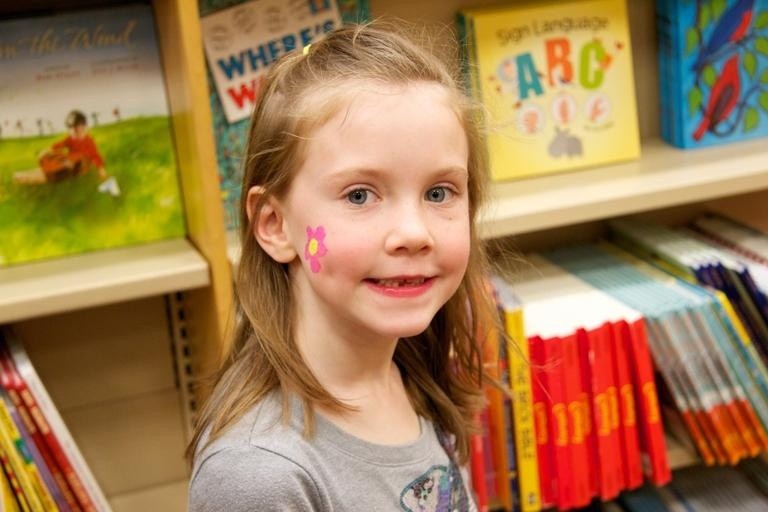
[654,0,768,149]
[455,0,643,181]
[444,213,767,512]
[1,338,114,512]
[0,3,188,269]
[198,0,371,231]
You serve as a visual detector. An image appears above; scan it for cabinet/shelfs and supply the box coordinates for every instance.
[0,1,767,511]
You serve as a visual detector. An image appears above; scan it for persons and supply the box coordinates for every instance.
[184,23,564,512]
[14,111,121,201]
